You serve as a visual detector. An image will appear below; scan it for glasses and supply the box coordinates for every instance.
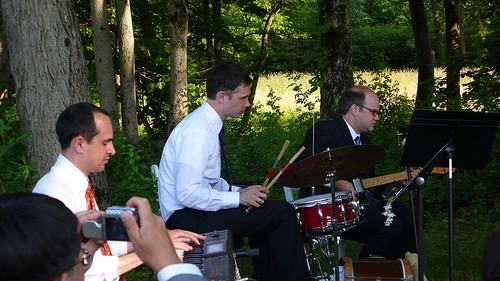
[57,247,93,281]
[348,102,382,117]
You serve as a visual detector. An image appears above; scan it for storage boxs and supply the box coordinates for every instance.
[352,259,403,281]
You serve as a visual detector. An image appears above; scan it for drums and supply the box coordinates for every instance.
[338,256,414,281]
[289,191,361,233]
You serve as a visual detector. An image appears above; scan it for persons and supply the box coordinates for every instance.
[158,64,309,281]
[0,191,208,281]
[299,85,426,259]
[32,103,206,281]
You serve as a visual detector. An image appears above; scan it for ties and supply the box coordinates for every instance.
[218,125,232,191]
[85,180,130,281]
[354,136,361,145]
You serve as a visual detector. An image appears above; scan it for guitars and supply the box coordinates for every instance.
[310,167,456,204]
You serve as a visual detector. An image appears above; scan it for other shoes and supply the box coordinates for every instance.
[405,252,427,281]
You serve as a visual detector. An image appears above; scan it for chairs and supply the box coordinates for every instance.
[283,187,345,261]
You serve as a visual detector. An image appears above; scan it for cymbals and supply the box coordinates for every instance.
[278,145,386,188]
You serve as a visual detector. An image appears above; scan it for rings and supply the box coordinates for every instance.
[258,198,262,204]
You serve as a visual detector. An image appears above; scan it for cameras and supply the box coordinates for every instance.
[81,206,139,241]
